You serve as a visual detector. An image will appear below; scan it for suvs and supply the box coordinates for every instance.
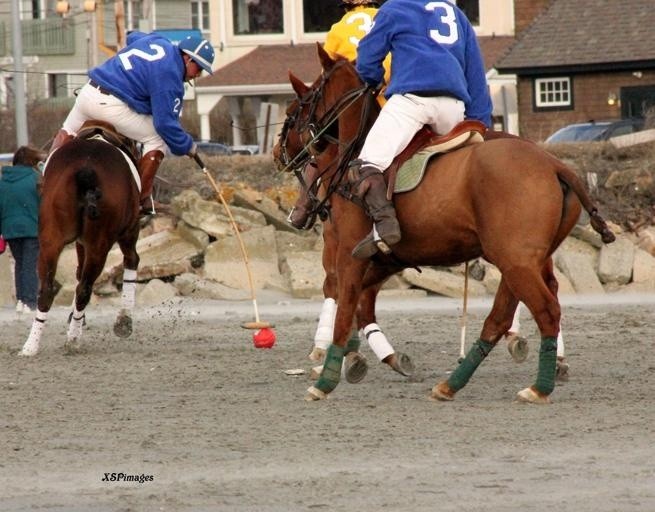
[544,118,643,142]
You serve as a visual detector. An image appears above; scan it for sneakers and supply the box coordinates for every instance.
[22,304,39,316]
[14,299,23,312]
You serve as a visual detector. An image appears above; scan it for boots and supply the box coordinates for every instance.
[139,149,165,213]
[351,165,402,261]
[48,128,75,160]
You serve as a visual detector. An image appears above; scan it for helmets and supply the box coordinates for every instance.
[178,35,216,77]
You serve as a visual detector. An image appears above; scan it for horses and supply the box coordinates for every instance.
[20,133,142,359]
[306,41,616,404]
[275,70,569,382]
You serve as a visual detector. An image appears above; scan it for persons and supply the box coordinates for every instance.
[1,146,42,313]
[321,0,391,83]
[350,0,491,260]
[43,32,214,212]
